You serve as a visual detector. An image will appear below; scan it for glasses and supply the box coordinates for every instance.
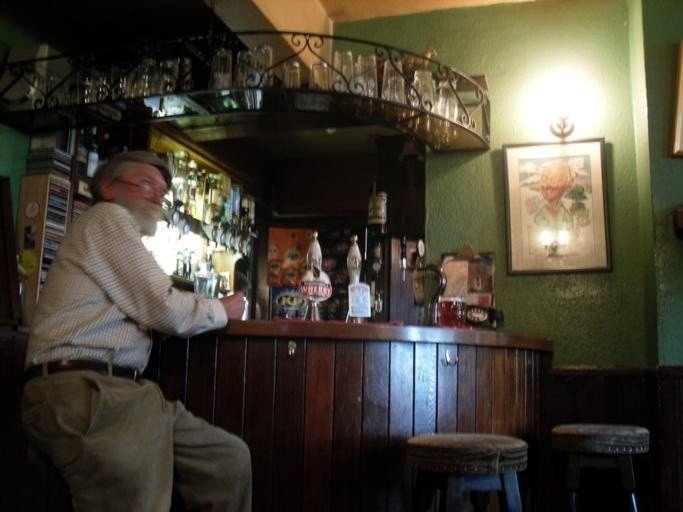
[113,179,171,197]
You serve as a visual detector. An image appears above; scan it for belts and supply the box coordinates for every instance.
[23,359,140,382]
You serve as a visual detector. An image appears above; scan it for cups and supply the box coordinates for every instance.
[382,59,407,103]
[334,50,354,93]
[253,44,273,86]
[309,62,329,91]
[206,48,232,89]
[193,272,221,300]
[283,62,300,88]
[72,57,191,102]
[437,81,457,121]
[356,53,379,97]
[413,70,433,111]
[234,51,254,86]
[438,295,466,327]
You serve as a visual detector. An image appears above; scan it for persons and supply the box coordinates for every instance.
[19,149,254,512]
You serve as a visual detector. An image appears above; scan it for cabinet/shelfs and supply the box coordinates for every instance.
[142,131,256,319]
[269,219,389,323]
[15,128,121,333]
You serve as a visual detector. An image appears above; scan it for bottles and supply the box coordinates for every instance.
[170,151,255,235]
[76,127,88,174]
[87,126,99,177]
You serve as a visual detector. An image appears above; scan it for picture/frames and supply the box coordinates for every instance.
[503,138,612,277]
[667,41,682,157]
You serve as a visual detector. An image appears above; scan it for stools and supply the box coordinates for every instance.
[403,432,527,511]
[552,423,650,511]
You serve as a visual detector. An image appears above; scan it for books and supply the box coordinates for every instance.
[71,176,93,229]
[23,146,98,179]
[40,237,61,294]
[45,175,70,237]
